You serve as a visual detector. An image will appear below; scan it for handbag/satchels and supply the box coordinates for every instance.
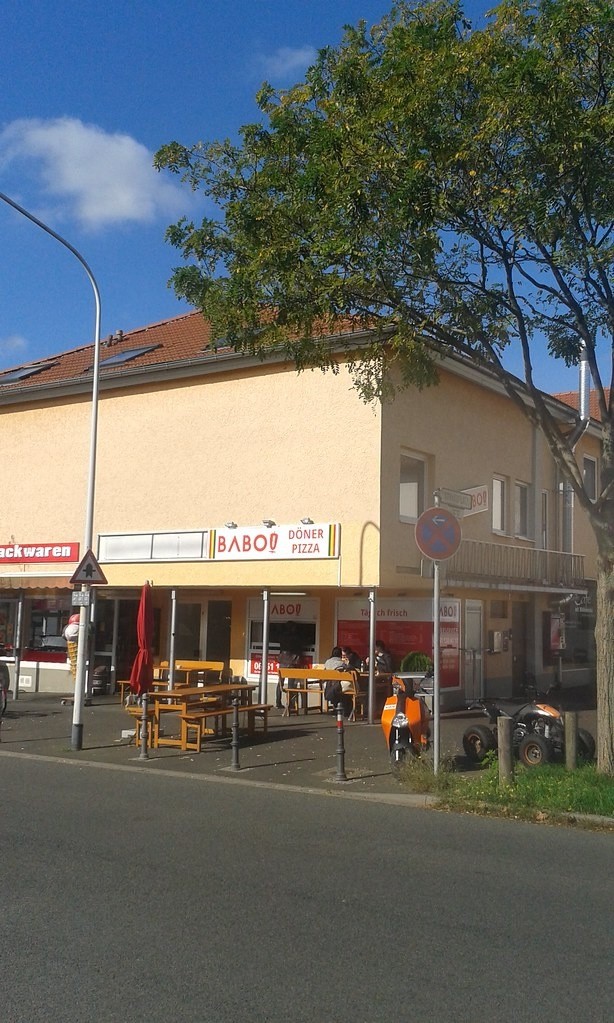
[324,666,345,702]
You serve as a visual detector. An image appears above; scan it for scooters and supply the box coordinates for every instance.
[376,656,432,781]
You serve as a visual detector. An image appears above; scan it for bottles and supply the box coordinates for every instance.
[360,661,365,672]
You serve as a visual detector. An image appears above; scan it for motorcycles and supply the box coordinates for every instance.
[461,681,596,769]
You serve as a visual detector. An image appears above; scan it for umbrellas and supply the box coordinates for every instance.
[129,580,154,739]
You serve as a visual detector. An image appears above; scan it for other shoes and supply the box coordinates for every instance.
[276,705,285,708]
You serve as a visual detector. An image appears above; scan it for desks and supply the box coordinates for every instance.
[360,672,392,719]
[396,672,430,694]
[152,664,213,684]
[146,684,258,751]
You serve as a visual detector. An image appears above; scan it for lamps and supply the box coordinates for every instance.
[300,517,314,525]
[225,521,238,529]
[261,519,276,528]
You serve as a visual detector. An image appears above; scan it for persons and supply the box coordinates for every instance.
[276,620,303,709]
[323,639,392,717]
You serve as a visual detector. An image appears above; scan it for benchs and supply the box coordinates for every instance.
[394,692,433,703]
[276,666,394,722]
[128,698,275,753]
[117,661,224,707]
